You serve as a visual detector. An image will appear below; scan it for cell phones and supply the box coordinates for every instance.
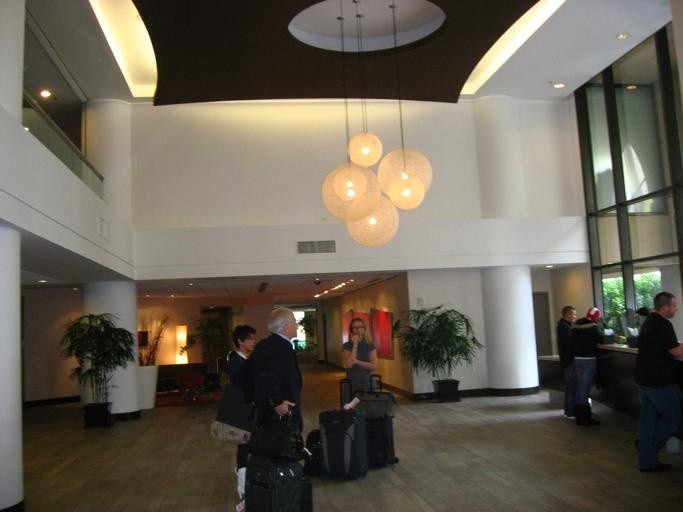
[351,331,355,337]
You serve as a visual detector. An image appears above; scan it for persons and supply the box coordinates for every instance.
[566,307,620,425]
[621,307,650,348]
[339,318,379,405]
[556,306,577,419]
[227,324,257,501]
[239,307,303,457]
[633,291,683,472]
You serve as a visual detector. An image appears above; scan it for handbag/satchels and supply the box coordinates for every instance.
[211,384,254,445]
[249,419,304,461]
[304,431,320,477]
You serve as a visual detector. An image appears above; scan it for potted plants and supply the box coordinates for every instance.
[55,311,137,430]
[390,301,484,402]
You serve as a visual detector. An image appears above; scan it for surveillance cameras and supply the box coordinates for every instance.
[314,278,321,285]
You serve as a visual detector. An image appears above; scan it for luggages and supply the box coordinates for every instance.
[244,461,311,512]
[318,375,398,477]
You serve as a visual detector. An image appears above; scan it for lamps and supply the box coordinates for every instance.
[174,325,188,364]
[319,0,434,250]
